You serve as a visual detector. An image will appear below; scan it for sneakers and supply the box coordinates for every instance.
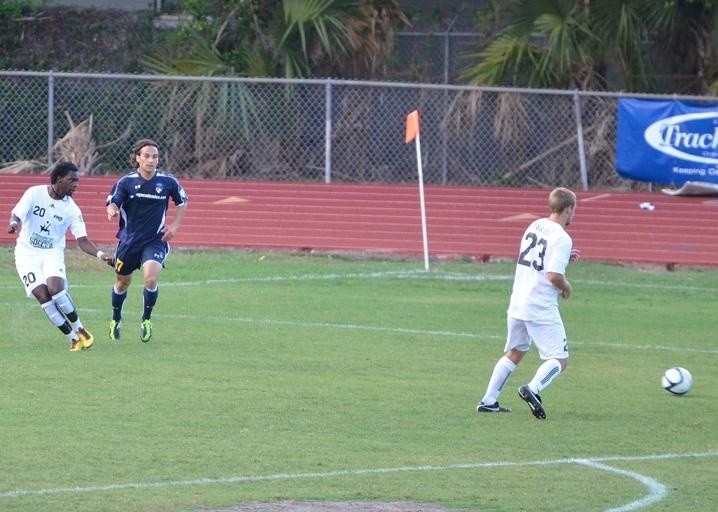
[141,319,153,343]
[75,327,94,349]
[517,383,547,421]
[476,400,512,413]
[109,317,122,341]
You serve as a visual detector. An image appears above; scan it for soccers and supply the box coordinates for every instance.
[662,367,692,395]
[639,202,655,212]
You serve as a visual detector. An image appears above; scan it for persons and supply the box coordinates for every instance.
[7,162,117,352]
[476,186,580,420]
[104,138,189,343]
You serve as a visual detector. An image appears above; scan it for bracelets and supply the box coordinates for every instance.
[9,221,18,225]
[97,250,103,260]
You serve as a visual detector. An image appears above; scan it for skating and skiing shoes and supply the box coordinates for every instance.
[68,338,83,353]
